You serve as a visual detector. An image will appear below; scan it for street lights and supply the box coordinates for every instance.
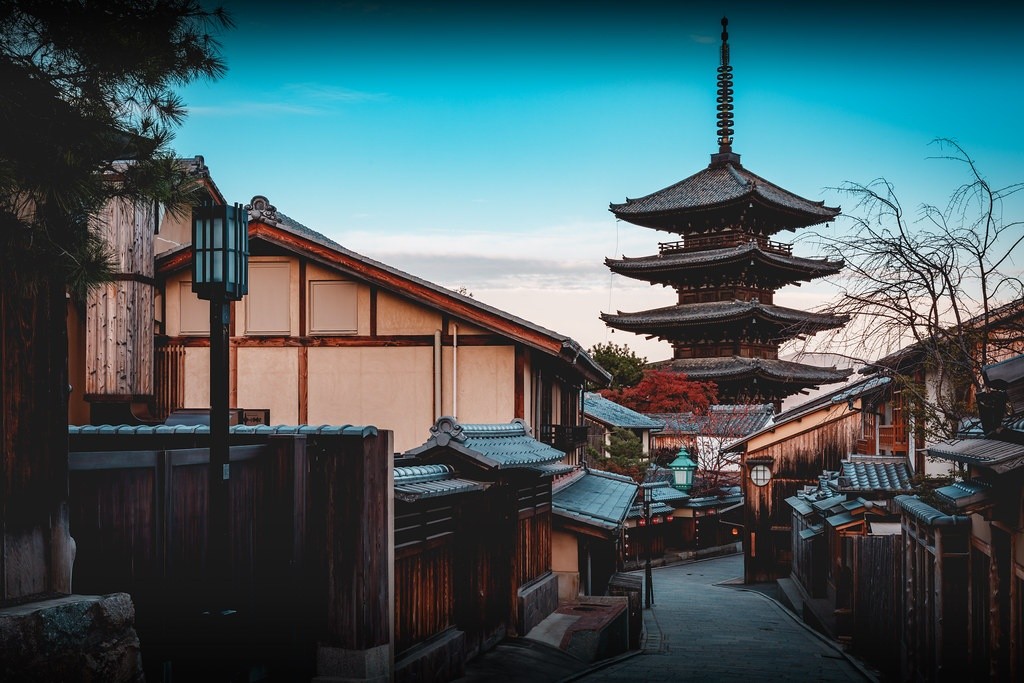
[643,486,652,608]
[190,198,250,683]
